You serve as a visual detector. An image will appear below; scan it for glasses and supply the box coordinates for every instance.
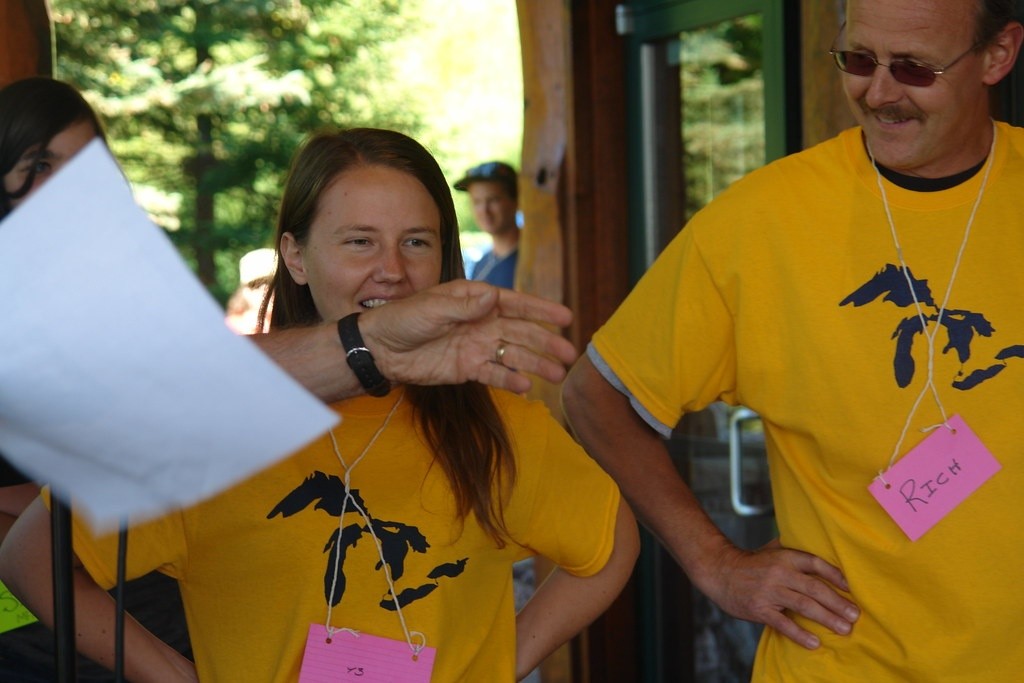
[466,164,498,178]
[830,19,990,87]
[247,277,275,289]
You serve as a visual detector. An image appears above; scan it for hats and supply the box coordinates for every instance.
[239,248,277,284]
[454,162,518,192]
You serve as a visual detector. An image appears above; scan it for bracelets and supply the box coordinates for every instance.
[338,312,391,397]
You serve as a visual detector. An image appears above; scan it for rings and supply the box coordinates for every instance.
[496,342,509,365]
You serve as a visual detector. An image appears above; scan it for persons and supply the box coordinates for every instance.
[224,249,281,334]
[0,1,106,683]
[0,129,641,682]
[0,278,576,489]
[563,0,1024,682]
[454,160,522,289]
